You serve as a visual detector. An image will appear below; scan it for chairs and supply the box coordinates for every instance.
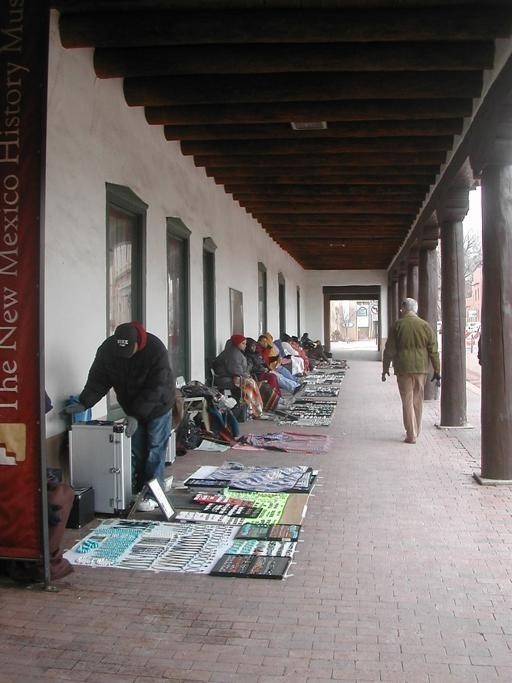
[176,376,211,433]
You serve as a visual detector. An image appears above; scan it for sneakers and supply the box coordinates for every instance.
[132,489,161,512]
[404,431,420,444]
[293,380,308,395]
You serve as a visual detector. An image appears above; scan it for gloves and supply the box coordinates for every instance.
[430,371,441,387]
[381,369,391,381]
[122,414,140,438]
[62,401,87,414]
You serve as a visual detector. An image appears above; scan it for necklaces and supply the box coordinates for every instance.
[65,516,236,575]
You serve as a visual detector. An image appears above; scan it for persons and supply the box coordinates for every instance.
[381,295,440,444]
[55,321,178,511]
[209,330,338,420]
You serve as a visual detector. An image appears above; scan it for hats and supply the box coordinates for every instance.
[245,338,258,351]
[113,320,139,358]
[230,334,246,346]
[263,332,273,347]
